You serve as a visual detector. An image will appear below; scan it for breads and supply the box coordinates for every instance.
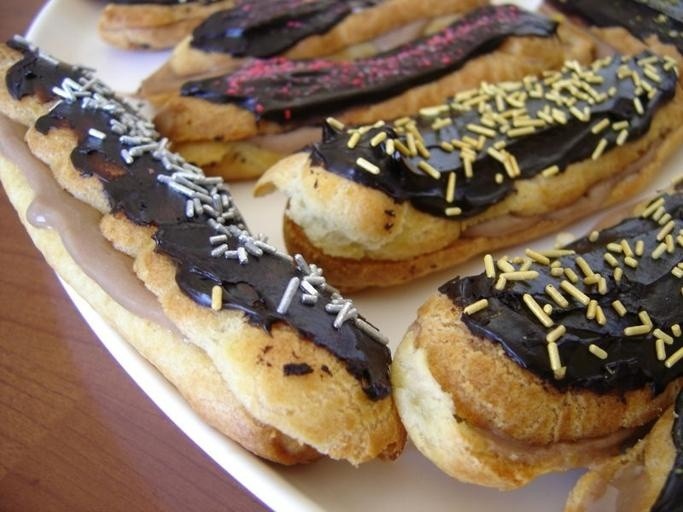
[95,1,241,52]
[250,46,683,290]
[0,34,407,469]
[134,0,487,107]
[385,175,682,491]
[536,1,683,89]
[564,383,682,512]
[151,3,563,183]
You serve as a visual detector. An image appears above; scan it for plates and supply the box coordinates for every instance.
[21,0,683,511]
